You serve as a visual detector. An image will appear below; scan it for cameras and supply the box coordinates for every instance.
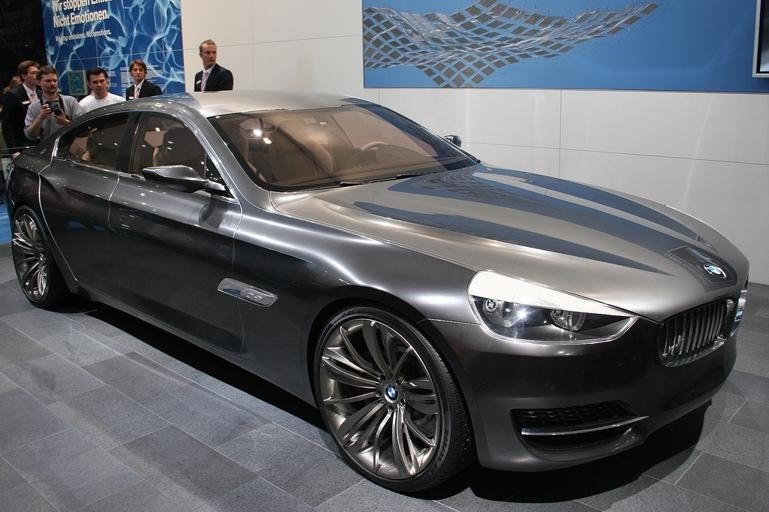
[45,100,60,116]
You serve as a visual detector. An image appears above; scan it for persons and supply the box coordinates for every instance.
[0,60,129,206]
[126,59,166,131]
[194,40,233,92]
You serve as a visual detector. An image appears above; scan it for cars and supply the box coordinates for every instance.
[5,90,749,495]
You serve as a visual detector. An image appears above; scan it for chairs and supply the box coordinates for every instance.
[268,119,317,181]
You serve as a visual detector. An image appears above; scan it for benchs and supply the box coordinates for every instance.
[80,123,266,194]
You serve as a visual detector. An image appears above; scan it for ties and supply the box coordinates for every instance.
[200,71,210,91]
[133,85,142,99]
[29,91,37,104]
